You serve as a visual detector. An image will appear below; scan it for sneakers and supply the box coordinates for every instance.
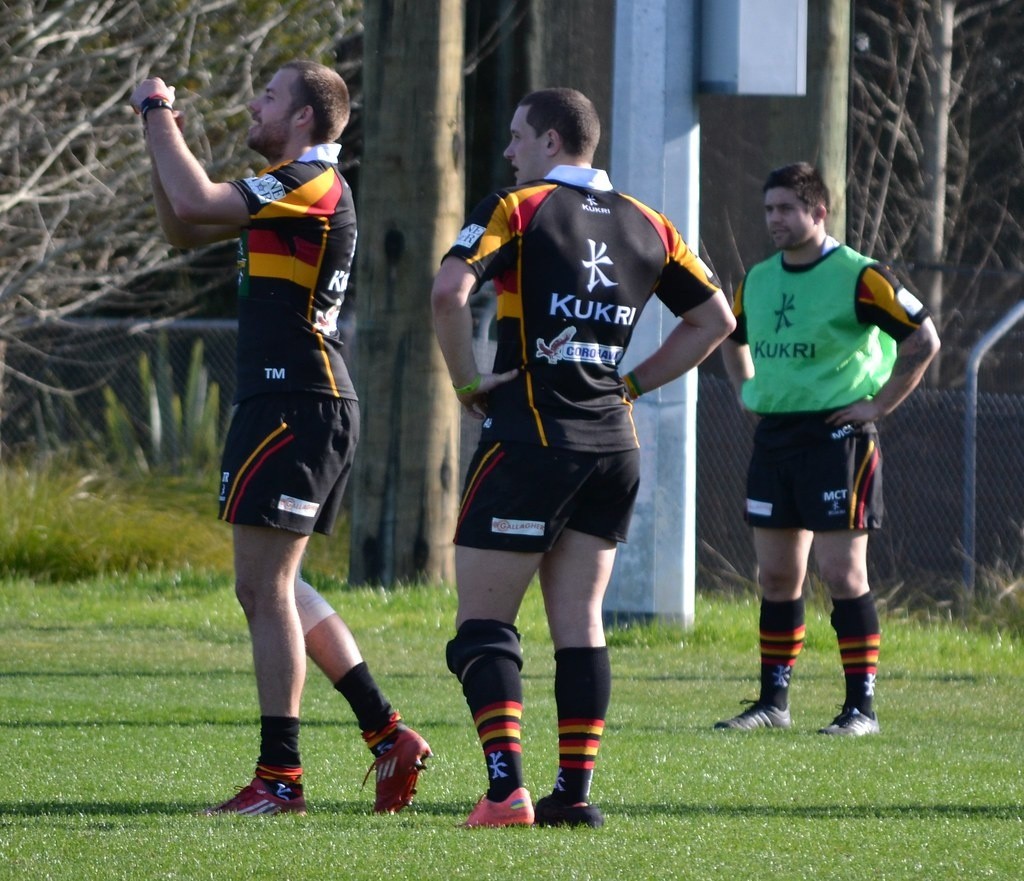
[534,791,605,828]
[712,699,790,731]
[360,728,435,814]
[461,787,536,827]
[819,701,880,738]
[203,779,307,821]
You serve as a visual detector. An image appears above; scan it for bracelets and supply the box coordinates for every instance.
[623,371,643,400]
[453,373,481,394]
[140,93,173,120]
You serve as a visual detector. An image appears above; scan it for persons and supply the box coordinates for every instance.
[715,162,942,737]
[130,59,433,815]
[431,89,737,829]
